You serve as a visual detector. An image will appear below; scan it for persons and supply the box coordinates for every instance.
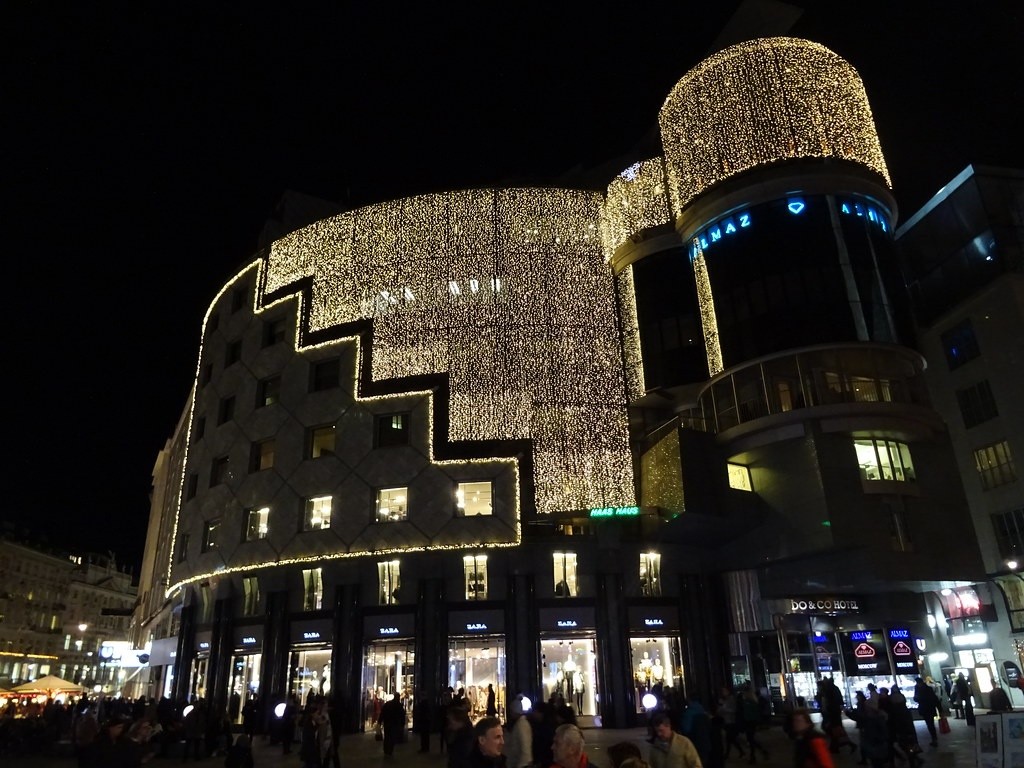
[363,685,600,768]
[0,692,261,768]
[608,674,1014,768]
[268,678,345,768]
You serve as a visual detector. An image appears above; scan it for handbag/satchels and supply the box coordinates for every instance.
[375,728,383,741]
[939,718,950,734]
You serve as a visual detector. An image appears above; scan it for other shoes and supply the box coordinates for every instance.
[747,759,757,764]
[417,750,429,753]
[850,744,857,755]
[856,760,868,765]
[383,754,393,761]
[761,756,769,761]
[738,752,745,758]
[918,759,925,768]
[831,748,841,754]
[929,742,938,747]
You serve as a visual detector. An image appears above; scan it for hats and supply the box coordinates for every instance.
[891,685,902,692]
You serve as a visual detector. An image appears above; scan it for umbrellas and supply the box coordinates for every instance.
[0,674,94,697]
